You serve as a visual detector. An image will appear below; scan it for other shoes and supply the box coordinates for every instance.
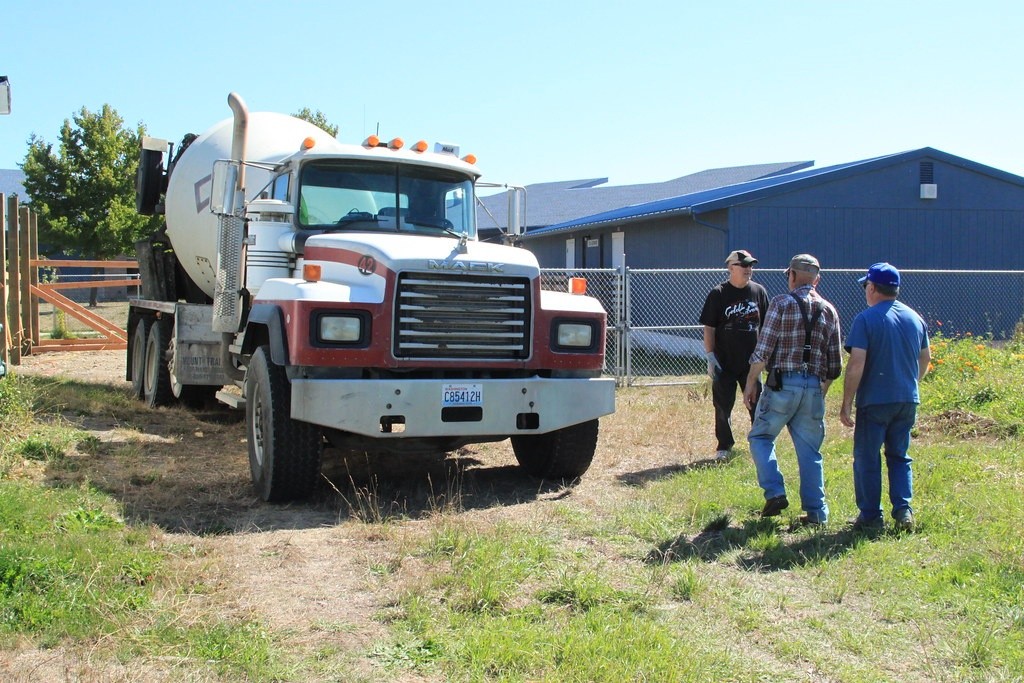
[797,515,819,525]
[716,450,731,463]
[895,509,913,532]
[762,496,789,517]
[846,517,882,532]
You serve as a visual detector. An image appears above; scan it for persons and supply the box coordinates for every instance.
[840,263,931,530]
[742,253,842,523]
[699,250,769,458]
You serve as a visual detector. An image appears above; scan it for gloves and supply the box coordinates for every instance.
[706,352,723,380]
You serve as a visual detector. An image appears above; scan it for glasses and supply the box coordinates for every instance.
[735,264,753,267]
[864,282,874,289]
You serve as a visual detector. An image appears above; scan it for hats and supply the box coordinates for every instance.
[725,250,759,265]
[858,262,901,287]
[782,254,819,274]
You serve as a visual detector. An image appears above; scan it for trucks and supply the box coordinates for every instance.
[126,91,615,502]
